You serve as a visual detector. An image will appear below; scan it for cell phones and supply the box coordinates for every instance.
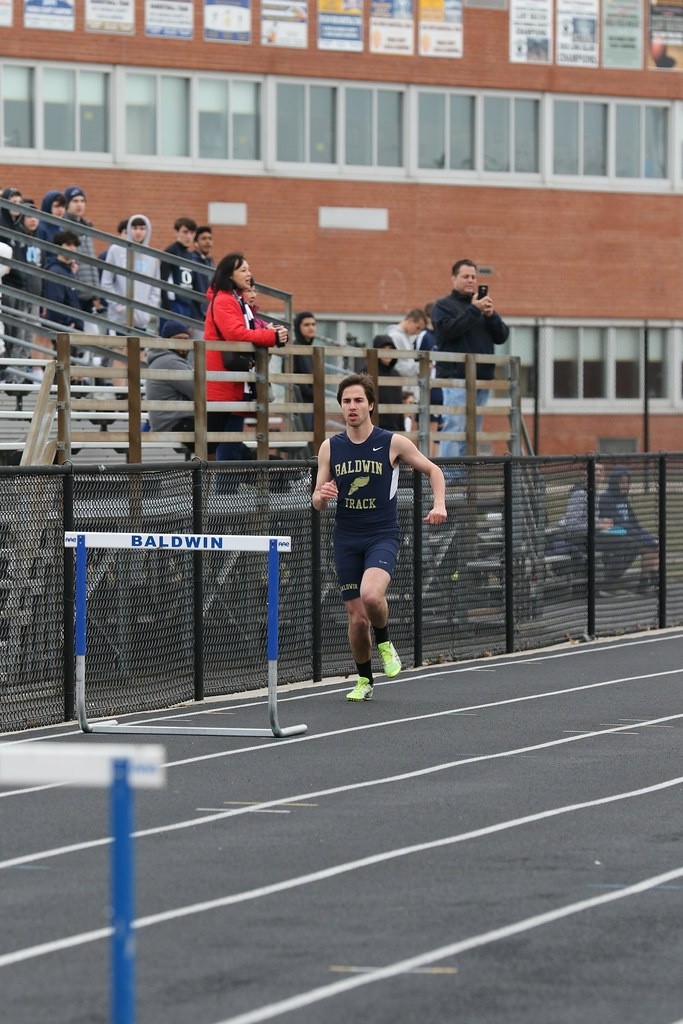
[478,285,487,300]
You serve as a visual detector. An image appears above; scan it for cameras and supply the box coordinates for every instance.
[274,325,289,347]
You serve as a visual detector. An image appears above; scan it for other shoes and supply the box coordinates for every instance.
[635,585,658,595]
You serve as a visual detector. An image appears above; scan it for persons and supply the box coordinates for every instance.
[0,187,669,598]
[312,374,447,703]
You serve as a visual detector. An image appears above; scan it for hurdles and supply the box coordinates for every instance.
[62,527,310,739]
[1,741,170,1024]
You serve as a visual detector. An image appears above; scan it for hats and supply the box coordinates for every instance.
[161,320,189,338]
[64,186,85,209]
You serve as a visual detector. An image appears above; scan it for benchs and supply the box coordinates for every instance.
[468,513,642,605]
[0,358,308,467]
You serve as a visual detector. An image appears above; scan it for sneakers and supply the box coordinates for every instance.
[377,640,401,678]
[345,676,373,701]
